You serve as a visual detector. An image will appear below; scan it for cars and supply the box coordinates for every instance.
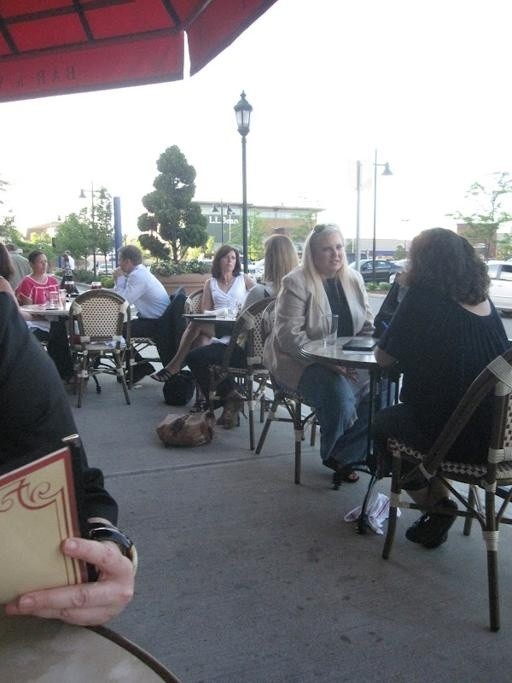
[108,252,117,260]
[203,246,409,287]
[481,257,512,311]
[97,262,113,276]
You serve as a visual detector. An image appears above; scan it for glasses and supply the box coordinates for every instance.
[216,393,248,430]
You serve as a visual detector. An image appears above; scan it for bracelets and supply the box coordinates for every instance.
[87,527,139,578]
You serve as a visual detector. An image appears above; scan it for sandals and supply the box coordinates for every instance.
[190,398,209,413]
[150,366,180,383]
[321,455,362,483]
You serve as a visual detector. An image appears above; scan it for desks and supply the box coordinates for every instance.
[0,603,183,683]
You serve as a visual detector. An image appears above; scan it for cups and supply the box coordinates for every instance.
[320,315,339,345]
[49,289,67,310]
[92,282,102,289]
[223,306,236,320]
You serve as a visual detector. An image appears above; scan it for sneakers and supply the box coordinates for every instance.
[405,499,459,550]
[124,362,156,386]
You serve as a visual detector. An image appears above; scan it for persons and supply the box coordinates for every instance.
[183,233,299,428]
[148,242,256,412]
[1,239,140,628]
[111,244,171,384]
[14,249,76,380]
[373,226,511,549]
[263,221,375,484]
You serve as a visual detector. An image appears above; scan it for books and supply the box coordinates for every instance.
[1,446,89,617]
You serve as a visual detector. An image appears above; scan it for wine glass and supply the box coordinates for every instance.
[64,281,74,302]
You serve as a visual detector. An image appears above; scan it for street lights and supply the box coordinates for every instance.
[209,199,235,248]
[233,88,254,273]
[78,182,110,277]
[55,214,68,222]
[371,159,394,288]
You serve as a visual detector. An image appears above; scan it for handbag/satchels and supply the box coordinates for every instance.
[156,410,217,447]
[162,369,196,406]
